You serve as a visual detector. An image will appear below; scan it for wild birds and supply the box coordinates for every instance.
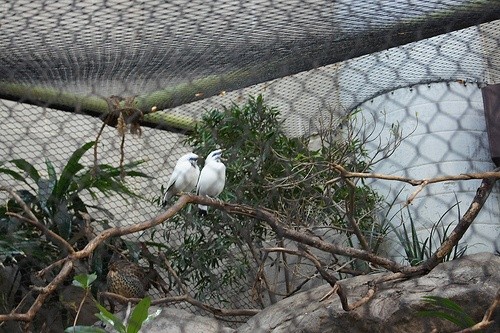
[162,152,200,206]
[196,148,229,217]
[106,260,157,306]
